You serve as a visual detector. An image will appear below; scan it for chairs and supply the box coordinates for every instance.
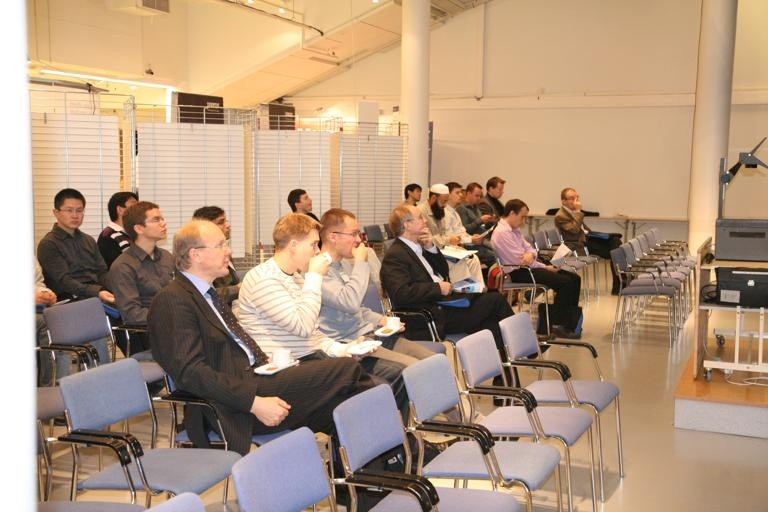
[159,373,290,446]
[46,294,165,446]
[331,383,521,510]
[359,221,696,349]
[56,356,242,505]
[497,310,624,503]
[144,491,205,512]
[36,419,145,512]
[227,426,432,512]
[31,342,88,480]
[454,329,597,512]
[400,352,564,510]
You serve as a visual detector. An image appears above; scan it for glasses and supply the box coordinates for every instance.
[193,238,232,251]
[332,228,363,238]
[138,216,165,225]
[406,214,424,223]
[58,207,86,215]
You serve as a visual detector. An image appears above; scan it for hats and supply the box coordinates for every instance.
[429,182,450,196]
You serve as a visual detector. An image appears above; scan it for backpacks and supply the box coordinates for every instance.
[486,261,521,308]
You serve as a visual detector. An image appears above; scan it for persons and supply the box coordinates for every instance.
[311,208,460,423]
[237,213,410,428]
[147,218,388,512]
[287,188,322,251]
[33,256,66,427]
[404,176,506,283]
[38,188,242,359]
[380,205,539,403]
[492,199,582,334]
[555,187,634,297]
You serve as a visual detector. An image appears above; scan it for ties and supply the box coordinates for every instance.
[205,286,271,369]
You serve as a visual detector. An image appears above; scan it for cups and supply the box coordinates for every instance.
[272,348,291,364]
[386,316,400,330]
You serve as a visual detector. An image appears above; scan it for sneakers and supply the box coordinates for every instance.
[549,324,581,339]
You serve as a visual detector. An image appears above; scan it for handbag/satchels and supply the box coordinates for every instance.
[584,228,624,240]
[536,301,584,340]
